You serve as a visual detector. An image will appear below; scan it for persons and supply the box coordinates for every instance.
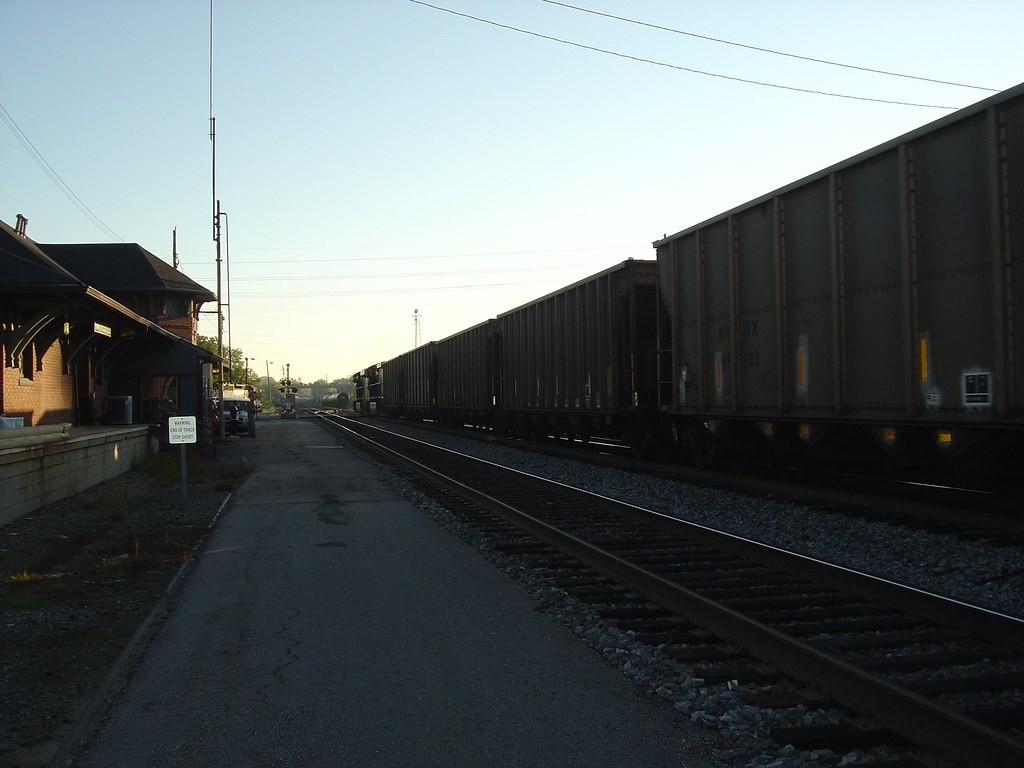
[229,401,240,439]
[248,399,257,438]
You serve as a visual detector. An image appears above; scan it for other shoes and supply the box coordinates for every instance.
[229,434,240,439]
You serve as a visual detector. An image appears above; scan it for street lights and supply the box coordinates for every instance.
[245,357,256,384]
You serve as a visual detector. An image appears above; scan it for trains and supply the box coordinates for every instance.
[321,392,350,407]
[351,83,1024,515]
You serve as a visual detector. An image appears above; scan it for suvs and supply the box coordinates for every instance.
[213,399,254,434]
